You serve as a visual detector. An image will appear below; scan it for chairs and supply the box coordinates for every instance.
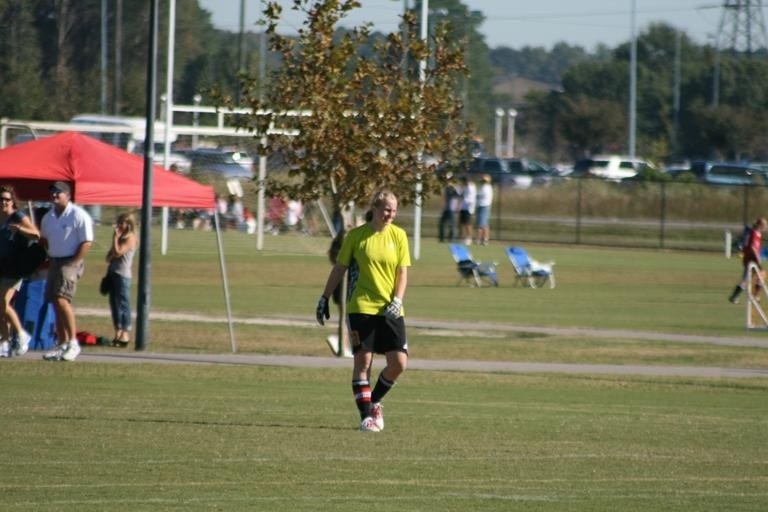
[506,246,557,289]
[449,243,499,288]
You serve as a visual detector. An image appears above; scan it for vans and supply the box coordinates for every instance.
[70,113,192,177]
[188,149,255,180]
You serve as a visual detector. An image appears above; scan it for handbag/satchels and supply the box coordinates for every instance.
[100,276,109,296]
[5,239,46,278]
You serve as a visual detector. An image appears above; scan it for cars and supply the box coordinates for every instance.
[434,155,768,188]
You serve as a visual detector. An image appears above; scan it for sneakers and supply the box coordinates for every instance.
[360,403,384,433]
[0,339,12,358]
[112,337,129,348]
[43,341,80,360]
[16,335,30,355]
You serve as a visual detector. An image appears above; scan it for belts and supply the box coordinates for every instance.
[49,256,73,262]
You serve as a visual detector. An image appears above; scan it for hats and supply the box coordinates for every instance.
[50,181,71,192]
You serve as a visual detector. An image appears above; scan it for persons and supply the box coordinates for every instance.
[437,175,462,244]
[456,175,476,245]
[39,181,94,360]
[727,217,767,303]
[149,193,362,238]
[475,175,494,246]
[328,223,363,310]
[0,184,41,356]
[105,213,139,346]
[315,191,413,434]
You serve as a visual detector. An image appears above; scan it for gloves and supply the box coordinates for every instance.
[316,296,330,326]
[384,298,403,320]
